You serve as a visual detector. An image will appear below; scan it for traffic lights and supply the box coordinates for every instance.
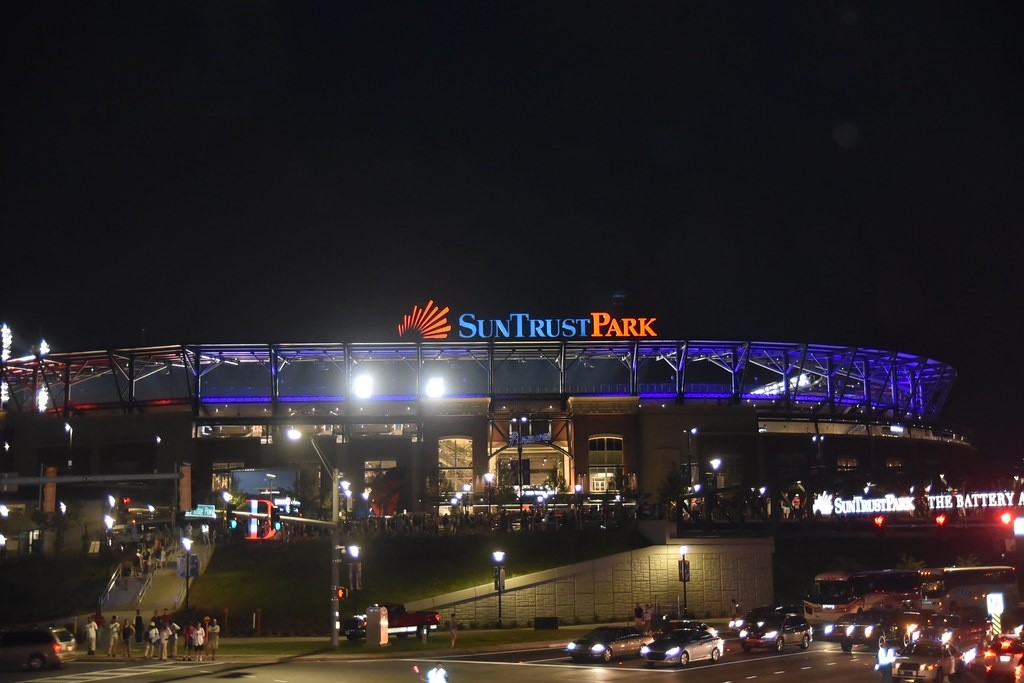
[338,587,348,599]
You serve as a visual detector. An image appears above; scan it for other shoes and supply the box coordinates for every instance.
[195,658,198,661]
[188,658,192,661]
[199,658,202,660]
[183,658,186,661]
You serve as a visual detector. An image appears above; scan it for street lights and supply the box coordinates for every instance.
[510,413,528,510]
[679,545,691,620]
[575,484,584,531]
[683,427,697,491]
[287,429,344,651]
[492,551,506,628]
[181,537,195,613]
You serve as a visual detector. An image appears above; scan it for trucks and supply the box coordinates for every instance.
[112,512,183,547]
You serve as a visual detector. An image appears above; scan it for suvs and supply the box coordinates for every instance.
[0,624,77,672]
[739,613,814,654]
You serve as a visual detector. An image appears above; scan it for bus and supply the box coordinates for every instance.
[920,566,1020,615]
[805,569,921,642]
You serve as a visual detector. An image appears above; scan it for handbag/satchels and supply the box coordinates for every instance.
[143,630,149,641]
[171,623,181,631]
[149,628,159,638]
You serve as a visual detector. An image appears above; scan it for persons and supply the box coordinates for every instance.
[134,520,210,576]
[731,599,738,621]
[635,603,643,625]
[228,491,806,536]
[450,613,457,647]
[84,607,220,662]
[428,662,448,683]
[643,603,653,632]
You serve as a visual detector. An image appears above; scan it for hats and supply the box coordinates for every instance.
[151,622,155,625]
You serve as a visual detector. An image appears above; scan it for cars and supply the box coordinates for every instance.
[877,612,1024,683]
[639,620,726,667]
[568,626,655,664]
[512,484,558,504]
[728,600,805,634]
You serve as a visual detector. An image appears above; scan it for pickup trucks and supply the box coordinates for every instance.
[343,603,441,643]
